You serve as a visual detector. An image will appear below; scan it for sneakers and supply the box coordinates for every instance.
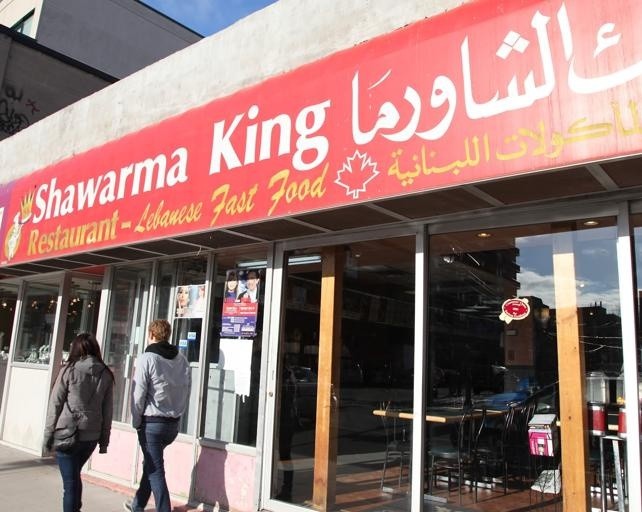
[124,500,134,512]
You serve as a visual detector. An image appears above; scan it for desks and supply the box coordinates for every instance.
[373,404,514,504]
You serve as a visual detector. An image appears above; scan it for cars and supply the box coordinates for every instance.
[279,365,338,423]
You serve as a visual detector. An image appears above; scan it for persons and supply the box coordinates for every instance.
[196,286,205,304]
[274,364,297,503]
[238,269,260,303]
[175,286,191,316]
[225,271,239,299]
[44,333,114,512]
[124,320,190,512]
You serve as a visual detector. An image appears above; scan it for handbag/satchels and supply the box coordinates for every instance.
[53,425,78,453]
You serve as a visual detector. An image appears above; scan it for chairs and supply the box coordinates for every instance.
[380,388,536,507]
